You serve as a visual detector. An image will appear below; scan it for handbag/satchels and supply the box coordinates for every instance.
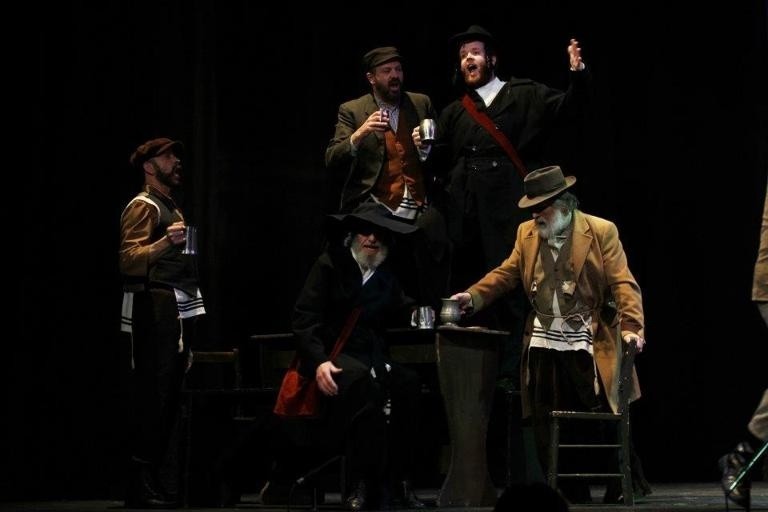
[274,365,322,414]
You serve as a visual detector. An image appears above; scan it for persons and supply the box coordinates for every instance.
[325,47,447,245]
[412,25,589,383]
[719,181,768,508]
[450,165,653,504]
[493,480,568,511]
[118,137,206,510]
[287,201,435,510]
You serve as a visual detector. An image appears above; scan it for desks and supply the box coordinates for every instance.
[389,328,511,507]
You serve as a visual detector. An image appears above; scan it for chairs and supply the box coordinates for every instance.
[193,348,275,506]
[250,333,346,512]
[547,341,639,506]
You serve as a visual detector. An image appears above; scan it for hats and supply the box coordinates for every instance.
[129,137,177,165]
[518,166,576,210]
[449,25,492,40]
[362,46,404,70]
[334,203,420,235]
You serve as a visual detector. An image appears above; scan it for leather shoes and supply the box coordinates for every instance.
[719,450,752,507]
[341,473,367,511]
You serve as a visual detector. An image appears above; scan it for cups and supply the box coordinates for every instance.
[181,226,199,255]
[410,306,435,330]
[419,118,438,145]
[376,110,390,133]
[439,298,463,328]
